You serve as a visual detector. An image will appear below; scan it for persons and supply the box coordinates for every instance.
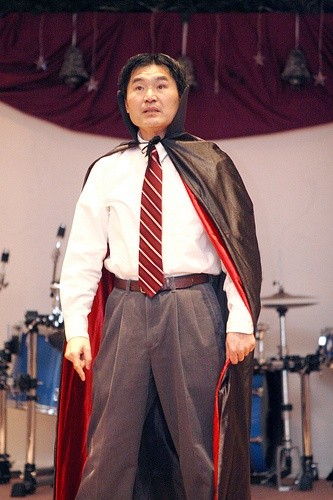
[51,49,264,500]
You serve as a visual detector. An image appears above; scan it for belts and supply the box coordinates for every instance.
[113,272,209,292]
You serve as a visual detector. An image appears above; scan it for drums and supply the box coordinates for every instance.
[249,370,268,482]
[5,310,65,417]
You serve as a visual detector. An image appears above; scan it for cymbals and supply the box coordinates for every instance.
[259,290,317,310]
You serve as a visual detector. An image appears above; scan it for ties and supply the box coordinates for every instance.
[138,140,165,299]
